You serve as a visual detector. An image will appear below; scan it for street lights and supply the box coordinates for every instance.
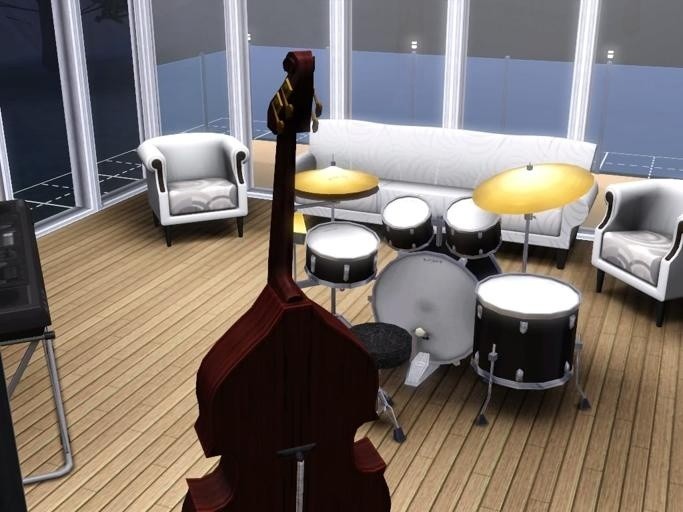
[410,41,419,122]
[599,48,616,141]
[500,54,511,121]
[247,33,251,44]
